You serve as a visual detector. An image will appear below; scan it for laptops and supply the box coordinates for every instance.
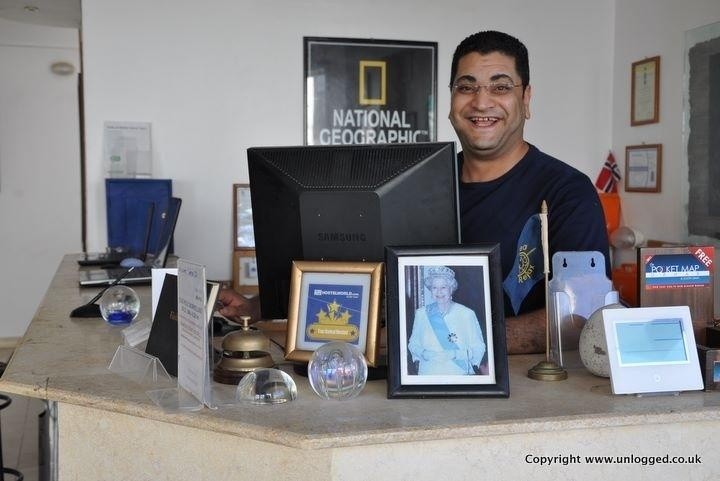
[78,201,155,266]
[80,196,182,286]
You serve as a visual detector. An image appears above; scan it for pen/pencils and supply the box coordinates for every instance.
[88,266,135,304]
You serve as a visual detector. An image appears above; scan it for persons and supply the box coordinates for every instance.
[407,266,486,375]
[216,30,611,355]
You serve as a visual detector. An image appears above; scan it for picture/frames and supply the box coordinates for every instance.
[601,303,705,400]
[232,249,260,295]
[283,256,384,372]
[301,34,441,146]
[230,181,258,250]
[623,143,663,193]
[703,348,720,392]
[630,55,661,127]
[384,240,511,402]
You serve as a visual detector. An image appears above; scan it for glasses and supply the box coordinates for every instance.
[448,81,526,95]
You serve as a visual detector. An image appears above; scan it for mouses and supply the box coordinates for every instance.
[120,257,146,268]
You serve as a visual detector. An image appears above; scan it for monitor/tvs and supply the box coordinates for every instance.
[246,140,462,382]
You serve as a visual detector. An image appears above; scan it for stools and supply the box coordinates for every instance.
[0,359,24,481]
